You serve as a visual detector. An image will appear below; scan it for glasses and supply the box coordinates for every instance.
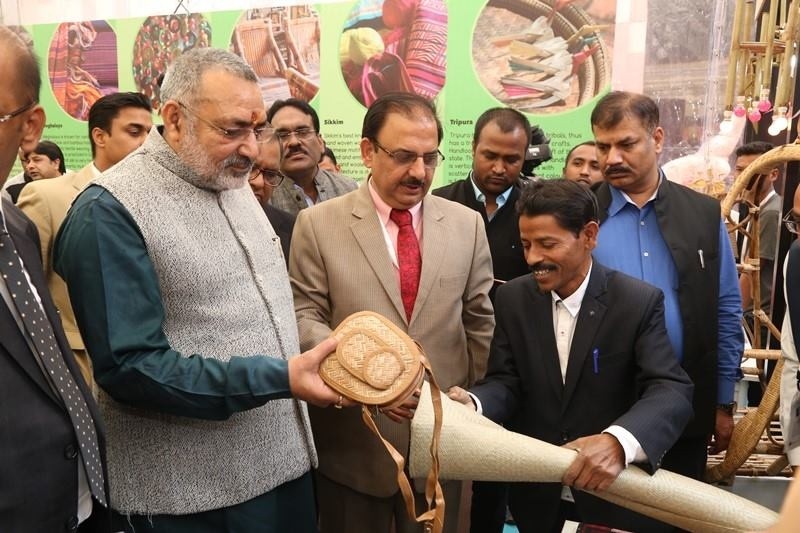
[372,137,445,169]
[277,128,318,139]
[248,166,284,188]
[782,208,800,234]
[176,98,275,143]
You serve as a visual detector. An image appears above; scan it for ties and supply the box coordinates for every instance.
[389,209,422,327]
[0,213,109,509]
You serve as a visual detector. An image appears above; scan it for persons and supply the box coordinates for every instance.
[290,91,495,533]
[432,106,542,533]
[6,140,65,204]
[778,182,800,477]
[12,91,153,404]
[733,141,783,406]
[563,141,604,190]
[248,120,298,273]
[442,179,695,532]
[2,139,74,195]
[583,90,746,533]
[53,46,364,532]
[318,147,341,173]
[267,98,359,217]
[0,22,112,533]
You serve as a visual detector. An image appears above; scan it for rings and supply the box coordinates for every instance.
[334,395,343,409]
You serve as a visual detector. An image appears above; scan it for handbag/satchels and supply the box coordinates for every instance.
[317,310,426,412]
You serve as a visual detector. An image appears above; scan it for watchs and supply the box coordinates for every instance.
[716,400,738,416]
